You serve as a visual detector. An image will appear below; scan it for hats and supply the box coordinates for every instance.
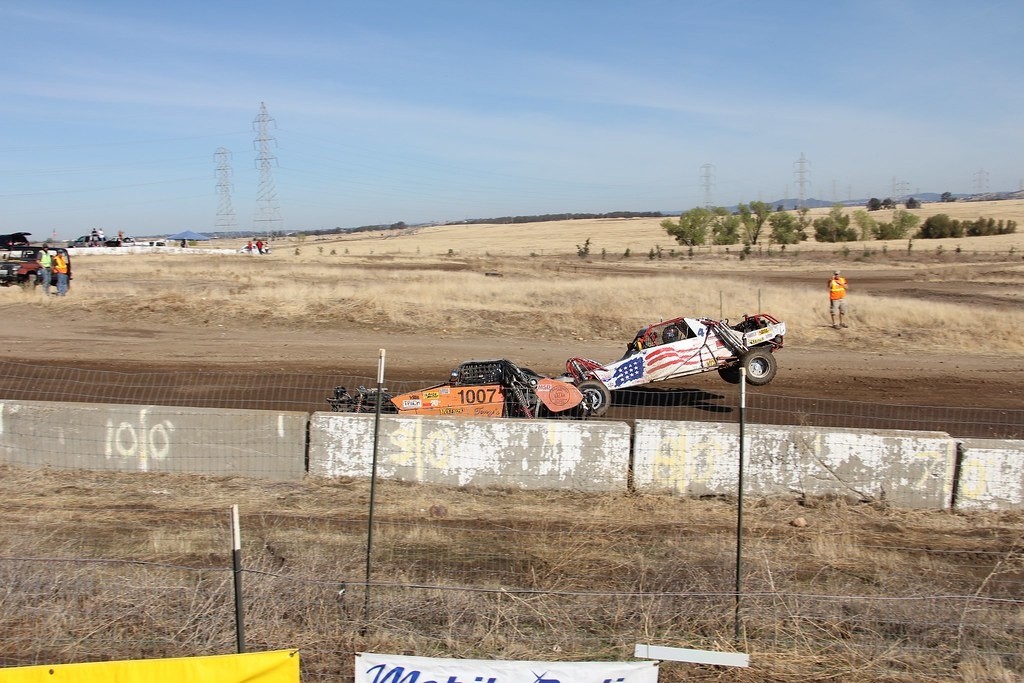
[834,270,840,275]
[57,249,63,253]
[42,242,48,248]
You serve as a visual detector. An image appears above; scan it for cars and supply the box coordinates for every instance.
[554,315,785,418]
[239,245,272,255]
[744,372,777,376]
[327,359,593,420]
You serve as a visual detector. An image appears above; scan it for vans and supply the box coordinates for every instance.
[0,232,73,289]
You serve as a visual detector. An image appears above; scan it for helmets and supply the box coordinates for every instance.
[661,326,678,343]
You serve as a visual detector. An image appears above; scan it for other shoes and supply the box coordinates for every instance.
[840,323,848,328]
[832,324,841,329]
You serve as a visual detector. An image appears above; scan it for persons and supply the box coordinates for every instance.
[662,326,678,345]
[829,271,849,329]
[247,239,269,255]
[52,249,68,296]
[118,228,125,247]
[87,227,106,247]
[36,243,52,296]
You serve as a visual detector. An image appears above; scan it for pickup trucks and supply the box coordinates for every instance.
[67,235,173,248]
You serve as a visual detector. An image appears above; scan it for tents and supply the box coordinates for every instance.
[167,230,211,248]
